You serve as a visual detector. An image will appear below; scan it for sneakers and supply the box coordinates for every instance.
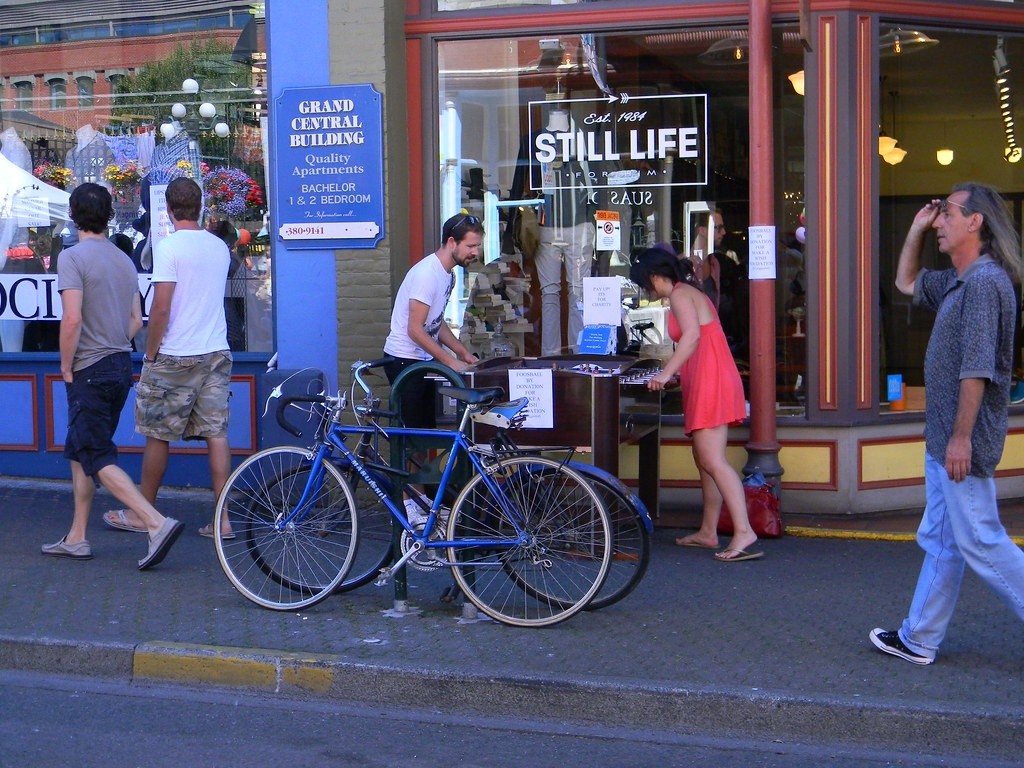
[41,535,92,559]
[871,628,935,666]
[418,499,461,520]
[137,516,186,570]
[391,507,427,527]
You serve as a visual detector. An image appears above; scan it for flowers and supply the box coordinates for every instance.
[32,158,264,222]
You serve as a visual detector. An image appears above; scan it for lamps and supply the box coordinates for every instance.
[883,89,906,166]
[936,122,954,168]
[698,38,778,67]
[788,52,804,96]
[878,27,939,57]
[879,74,897,154]
[991,37,1022,164]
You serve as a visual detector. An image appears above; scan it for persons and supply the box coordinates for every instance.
[141,121,204,269]
[0,126,32,272]
[869,182,1024,665]
[630,248,765,562]
[103,171,235,538]
[384,210,486,526]
[677,210,749,355]
[52,124,115,250]
[501,109,598,356]
[43,183,185,567]
[553,363,612,373]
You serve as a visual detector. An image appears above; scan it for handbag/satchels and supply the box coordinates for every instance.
[716,474,783,537]
[502,203,540,267]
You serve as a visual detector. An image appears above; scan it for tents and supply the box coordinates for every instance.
[0,152,72,223]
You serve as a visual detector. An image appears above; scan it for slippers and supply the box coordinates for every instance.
[103,509,149,532]
[200,524,237,539]
[712,547,764,561]
[676,534,721,549]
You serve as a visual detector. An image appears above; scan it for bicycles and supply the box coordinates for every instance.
[245,358,653,609]
[211,383,612,628]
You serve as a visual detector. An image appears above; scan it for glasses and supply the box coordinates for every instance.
[939,198,970,212]
[633,255,641,265]
[714,225,727,232]
[452,215,482,230]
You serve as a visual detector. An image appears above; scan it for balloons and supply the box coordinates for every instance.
[795,208,805,244]
